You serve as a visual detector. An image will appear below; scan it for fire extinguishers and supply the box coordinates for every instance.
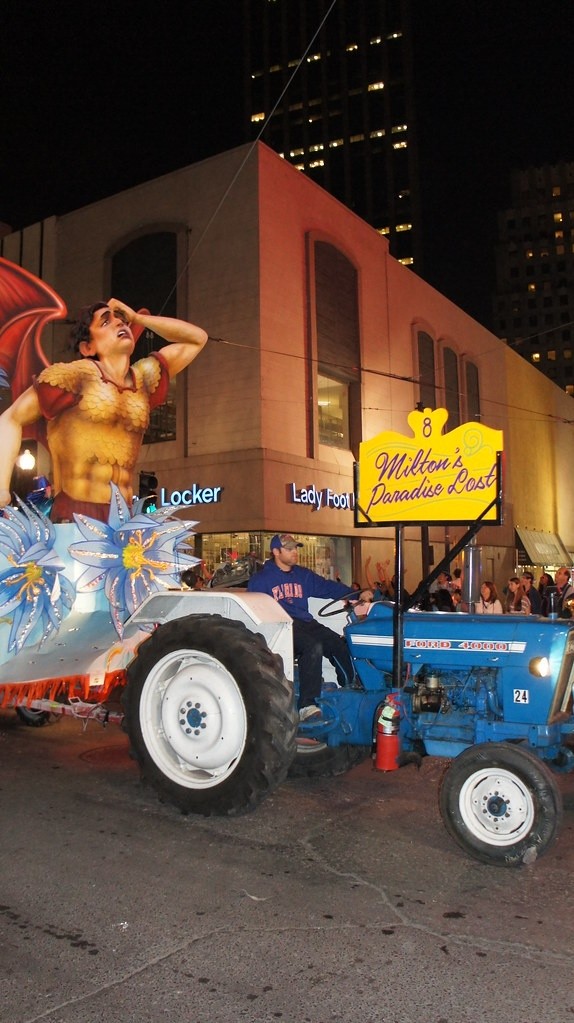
[370,693,405,771]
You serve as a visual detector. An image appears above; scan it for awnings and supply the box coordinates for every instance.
[514,528,574,566]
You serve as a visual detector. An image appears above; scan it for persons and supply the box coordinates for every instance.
[333,555,463,612]
[554,567,574,612]
[506,576,531,614]
[249,533,374,722]
[521,571,542,613]
[473,578,503,614]
[538,573,557,613]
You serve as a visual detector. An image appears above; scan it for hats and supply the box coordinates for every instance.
[270,534,304,549]
[442,563,453,582]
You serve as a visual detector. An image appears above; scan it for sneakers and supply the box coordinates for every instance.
[299,705,322,721]
